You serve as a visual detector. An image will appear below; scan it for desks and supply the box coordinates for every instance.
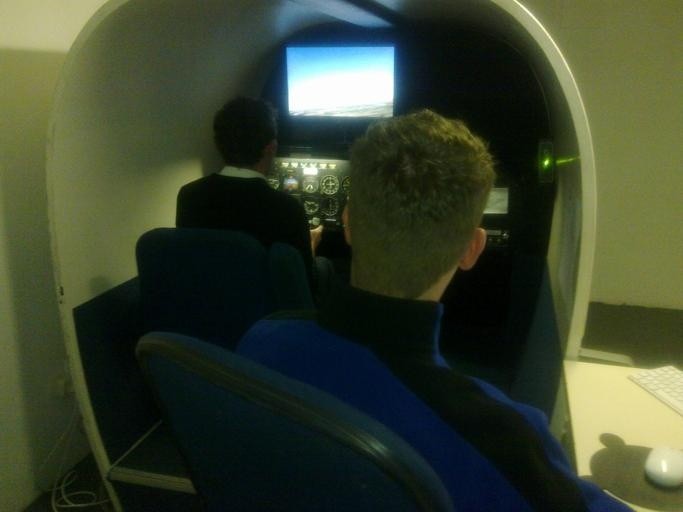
[550,303,683,512]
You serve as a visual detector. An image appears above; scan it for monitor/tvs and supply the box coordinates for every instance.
[281,41,399,119]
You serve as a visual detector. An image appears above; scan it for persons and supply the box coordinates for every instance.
[173,95,344,341]
[228,109,637,511]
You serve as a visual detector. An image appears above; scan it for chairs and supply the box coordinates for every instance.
[134,330,455,512]
[135,228,320,345]
